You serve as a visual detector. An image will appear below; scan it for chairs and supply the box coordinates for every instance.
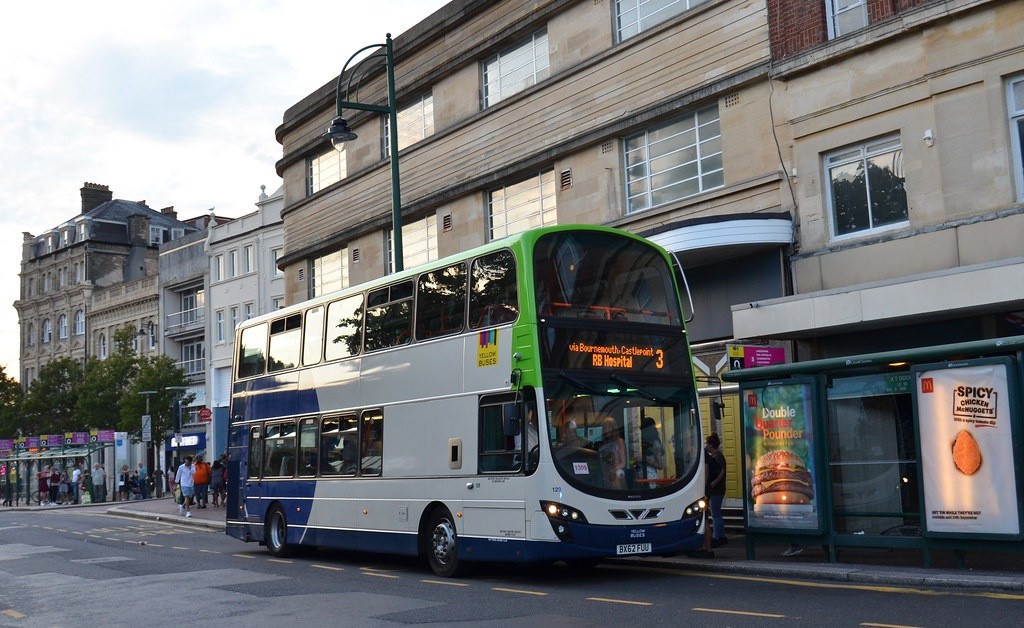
[394,304,506,347]
[576,310,628,320]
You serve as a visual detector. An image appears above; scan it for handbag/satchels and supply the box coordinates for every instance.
[174,484,183,504]
[119,481,124,485]
[223,469,228,481]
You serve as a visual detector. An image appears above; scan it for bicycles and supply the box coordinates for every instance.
[31,483,67,505]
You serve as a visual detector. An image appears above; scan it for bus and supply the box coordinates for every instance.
[224,223,727,579]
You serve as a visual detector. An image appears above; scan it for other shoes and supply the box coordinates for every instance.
[781,544,803,556]
[179,505,185,515]
[186,511,192,517]
[40,501,46,506]
[710,538,729,547]
[49,502,58,505]
[62,502,72,505]
[198,505,206,509]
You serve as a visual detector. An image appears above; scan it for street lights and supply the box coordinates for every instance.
[321,32,404,273]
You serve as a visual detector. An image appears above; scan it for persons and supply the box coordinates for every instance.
[514,407,538,469]
[636,416,664,489]
[119,453,228,517]
[18,463,107,507]
[598,418,627,489]
[557,420,593,448]
[672,431,728,557]
[782,544,803,556]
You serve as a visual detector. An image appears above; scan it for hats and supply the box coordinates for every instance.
[196,454,203,459]
[186,456,193,461]
